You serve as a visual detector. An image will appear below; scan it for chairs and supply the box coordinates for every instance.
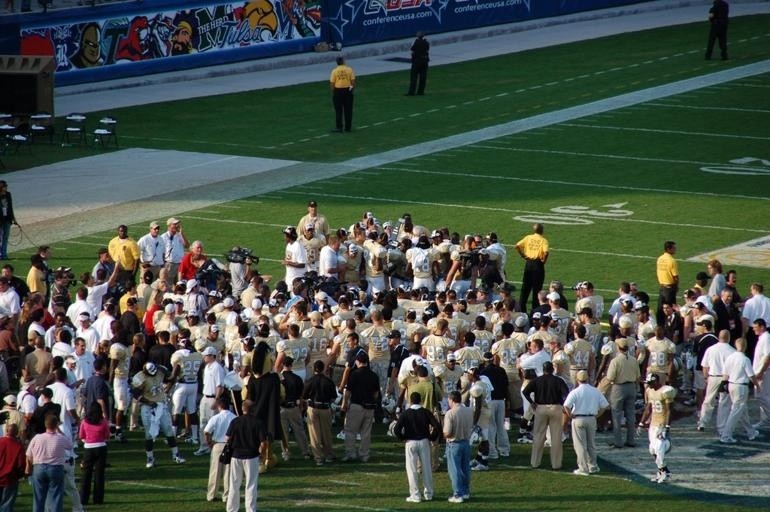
[0,111,119,156]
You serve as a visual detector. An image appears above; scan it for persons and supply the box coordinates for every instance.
[330,57,356,133]
[394,392,440,503]
[0,416,73,511]
[704,0,729,60]
[442,391,473,502]
[0,180,18,260]
[204,400,267,512]
[636,374,675,483]
[79,403,110,504]
[404,31,431,97]
[1,203,770,475]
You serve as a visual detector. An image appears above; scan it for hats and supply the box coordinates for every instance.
[282,226,297,238]
[429,229,443,239]
[550,312,560,321]
[150,220,160,229]
[3,394,17,406]
[515,316,528,327]
[367,217,381,227]
[447,353,456,362]
[65,357,77,363]
[166,218,180,225]
[546,292,560,300]
[336,227,351,239]
[174,298,184,303]
[79,315,89,321]
[314,291,328,301]
[27,330,44,340]
[208,324,220,333]
[21,380,36,391]
[580,281,593,289]
[354,222,366,230]
[407,309,416,319]
[143,361,158,376]
[176,338,191,348]
[643,372,658,383]
[631,300,647,311]
[386,330,401,338]
[483,232,498,242]
[187,309,198,317]
[546,336,560,343]
[577,307,592,315]
[620,298,634,309]
[164,303,176,313]
[615,337,628,350]
[251,298,262,309]
[478,249,489,255]
[206,290,220,299]
[364,210,374,219]
[268,298,280,307]
[696,272,711,280]
[696,319,712,327]
[576,370,588,381]
[307,310,321,320]
[383,222,395,228]
[305,223,314,230]
[309,201,317,208]
[57,266,72,272]
[496,282,515,293]
[464,367,479,378]
[236,314,249,323]
[185,278,200,293]
[241,336,255,345]
[530,311,542,320]
[413,357,427,368]
[422,310,434,321]
[223,298,235,307]
[200,346,217,356]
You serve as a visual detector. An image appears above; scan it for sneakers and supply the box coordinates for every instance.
[574,468,590,476]
[177,432,192,439]
[114,432,126,441]
[748,430,759,440]
[405,496,421,503]
[194,447,210,456]
[447,495,464,502]
[185,438,199,444]
[589,466,601,473]
[517,437,533,444]
[172,455,187,464]
[336,430,345,439]
[463,494,470,499]
[657,471,672,483]
[683,398,697,405]
[471,463,489,471]
[146,455,156,467]
[469,458,479,466]
[129,424,143,432]
[651,471,662,482]
[424,495,433,500]
[719,434,737,443]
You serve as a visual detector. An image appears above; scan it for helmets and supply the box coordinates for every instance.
[387,420,405,436]
[333,385,343,405]
[331,402,341,412]
[665,439,672,453]
[673,357,683,371]
[468,425,482,447]
[381,397,396,413]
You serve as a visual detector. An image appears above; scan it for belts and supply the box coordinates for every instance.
[710,375,722,377]
[730,382,747,385]
[206,395,216,398]
[334,363,346,367]
[575,414,595,417]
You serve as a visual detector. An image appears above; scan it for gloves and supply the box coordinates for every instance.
[637,423,644,437]
[663,425,670,439]
